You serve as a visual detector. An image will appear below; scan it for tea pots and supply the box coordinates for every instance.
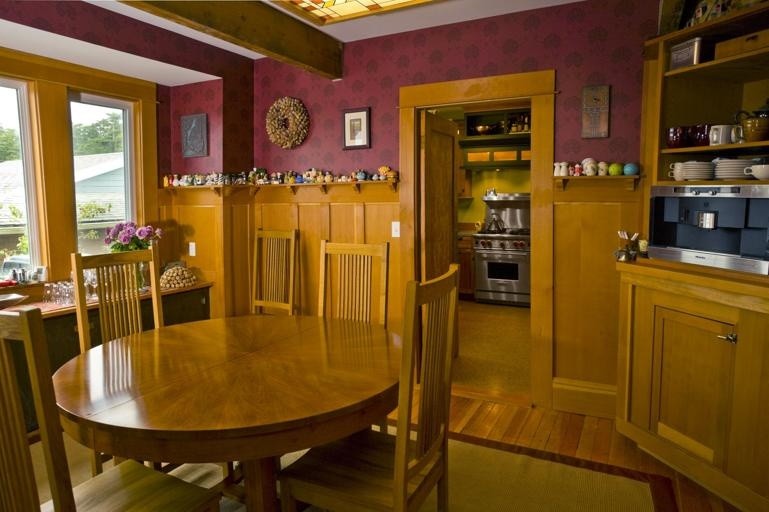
[709,109,768,146]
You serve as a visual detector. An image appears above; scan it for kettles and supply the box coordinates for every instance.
[485,214,504,236]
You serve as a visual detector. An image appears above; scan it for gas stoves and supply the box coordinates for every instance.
[479,225,530,234]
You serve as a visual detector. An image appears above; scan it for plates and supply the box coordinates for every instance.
[670,158,755,181]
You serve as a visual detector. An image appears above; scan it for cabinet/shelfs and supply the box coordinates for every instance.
[612,278,769,508]
[657,0,769,185]
[8,287,211,432]
[461,113,531,167]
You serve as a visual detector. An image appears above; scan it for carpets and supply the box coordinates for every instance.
[24,414,682,512]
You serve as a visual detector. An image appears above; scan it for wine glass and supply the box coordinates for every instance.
[75,263,106,303]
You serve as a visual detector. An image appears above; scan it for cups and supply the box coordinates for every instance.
[44,278,80,306]
[745,164,769,182]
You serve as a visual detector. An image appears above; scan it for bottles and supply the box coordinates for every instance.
[512,114,529,132]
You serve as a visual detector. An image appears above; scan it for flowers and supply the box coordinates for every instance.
[263,96,311,149]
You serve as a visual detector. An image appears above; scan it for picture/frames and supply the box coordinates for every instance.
[341,107,372,152]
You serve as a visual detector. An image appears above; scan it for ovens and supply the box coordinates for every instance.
[474,237,529,306]
